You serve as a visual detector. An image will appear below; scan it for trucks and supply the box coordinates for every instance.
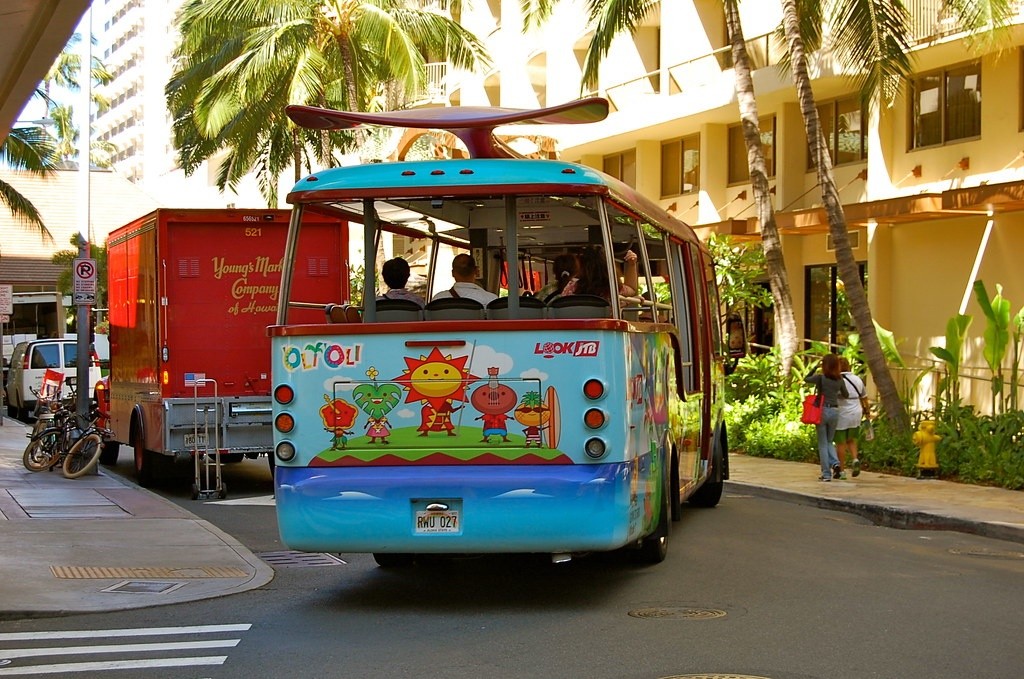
[93,206,354,482]
[4,291,67,381]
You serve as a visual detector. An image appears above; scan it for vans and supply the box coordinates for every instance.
[3,339,103,421]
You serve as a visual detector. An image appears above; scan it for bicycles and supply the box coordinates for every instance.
[22,376,112,478]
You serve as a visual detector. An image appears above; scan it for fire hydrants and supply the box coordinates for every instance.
[910,421,945,482]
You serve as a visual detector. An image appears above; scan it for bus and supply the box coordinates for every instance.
[265,95,746,570]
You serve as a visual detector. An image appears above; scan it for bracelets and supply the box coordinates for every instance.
[866,412,870,415]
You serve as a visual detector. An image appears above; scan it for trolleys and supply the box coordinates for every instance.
[193,380,228,501]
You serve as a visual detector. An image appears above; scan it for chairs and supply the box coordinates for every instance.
[377,296,613,322]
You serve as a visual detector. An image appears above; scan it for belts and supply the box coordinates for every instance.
[828,404,838,407]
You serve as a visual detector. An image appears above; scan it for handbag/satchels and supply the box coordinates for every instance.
[800,375,825,425]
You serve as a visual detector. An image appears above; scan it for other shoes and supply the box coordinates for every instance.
[833,464,841,479]
[818,475,831,481]
[840,471,847,480]
[852,459,860,477]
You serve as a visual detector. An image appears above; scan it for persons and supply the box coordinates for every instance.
[431,253,499,310]
[639,292,667,323]
[729,320,743,349]
[375,257,425,309]
[803,353,849,482]
[833,357,870,480]
[534,245,644,320]
